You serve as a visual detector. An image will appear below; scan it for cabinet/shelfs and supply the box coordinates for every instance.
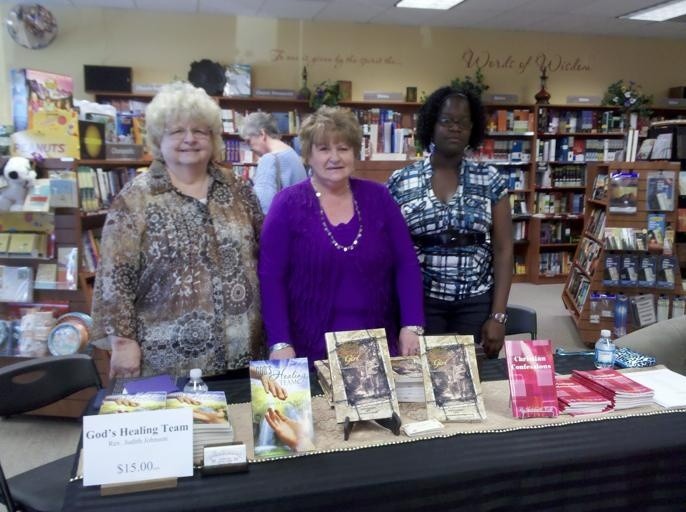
[79,90,685,283]
[1,157,153,422]
[562,163,685,348]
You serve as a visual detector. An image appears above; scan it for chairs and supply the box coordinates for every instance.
[612,316,686,376]
[0,354,108,512]
[504,305,538,349]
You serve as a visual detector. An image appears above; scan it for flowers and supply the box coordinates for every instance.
[604,79,652,106]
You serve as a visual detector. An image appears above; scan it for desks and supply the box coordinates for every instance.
[2,354,686,512]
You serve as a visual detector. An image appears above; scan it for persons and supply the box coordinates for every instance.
[237,110,309,216]
[88,78,267,382]
[257,100,429,363]
[251,366,290,402]
[263,404,307,450]
[380,83,518,360]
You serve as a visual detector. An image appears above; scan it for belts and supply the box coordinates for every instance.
[421,231,487,248]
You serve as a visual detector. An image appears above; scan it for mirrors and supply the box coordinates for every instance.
[5,3,58,51]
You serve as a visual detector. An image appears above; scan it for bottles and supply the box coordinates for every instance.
[594,328,616,369]
[184,370,208,392]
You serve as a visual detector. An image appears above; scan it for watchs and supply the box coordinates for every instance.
[488,312,510,325]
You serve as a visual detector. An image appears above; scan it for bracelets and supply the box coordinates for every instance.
[269,341,293,350]
[407,324,426,335]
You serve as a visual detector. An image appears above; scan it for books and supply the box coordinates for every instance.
[463,106,683,317]
[247,357,316,460]
[92,374,234,498]
[322,327,401,423]
[389,356,426,404]
[217,107,418,185]
[0,66,149,305]
[312,358,333,408]
[502,339,656,419]
[109,164,145,203]
[417,332,489,425]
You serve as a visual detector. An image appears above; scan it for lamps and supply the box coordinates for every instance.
[535,66,551,105]
[394,0,466,12]
[614,1,685,22]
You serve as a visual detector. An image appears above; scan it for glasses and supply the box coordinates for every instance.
[438,116,472,129]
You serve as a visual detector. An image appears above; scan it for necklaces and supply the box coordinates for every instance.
[313,182,365,255]
